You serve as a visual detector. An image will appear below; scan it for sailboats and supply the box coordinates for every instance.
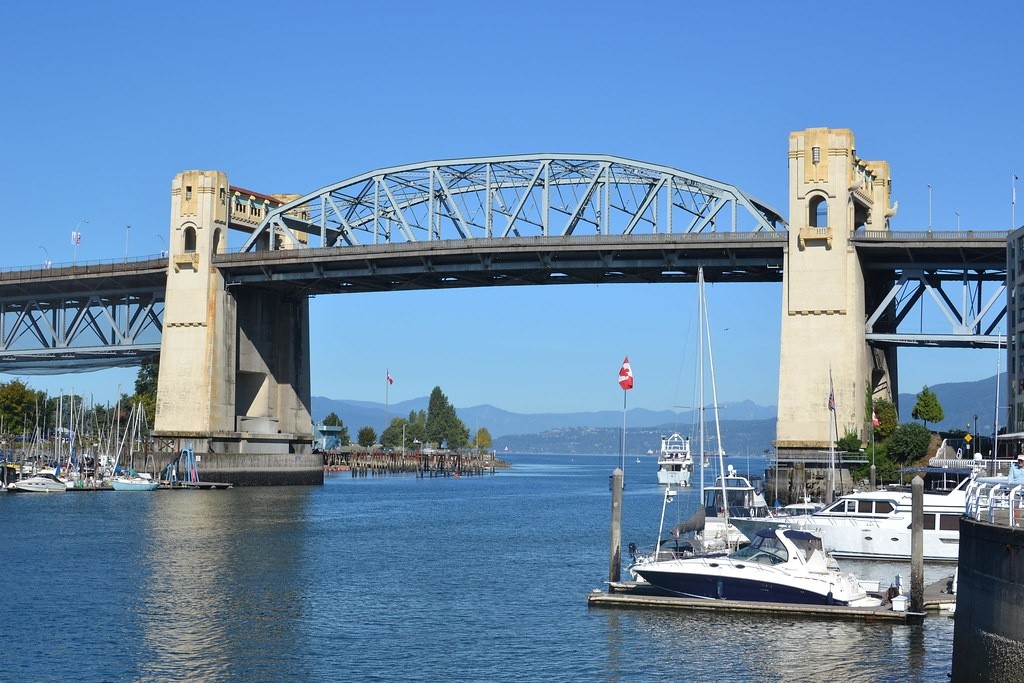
[0,387,234,493]
[629,264,897,609]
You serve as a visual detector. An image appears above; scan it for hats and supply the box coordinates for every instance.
[1017,455,1024,460]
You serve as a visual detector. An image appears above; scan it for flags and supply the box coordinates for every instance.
[828,379,835,411]
[618,357,634,391]
[872,405,880,428]
[386,369,394,385]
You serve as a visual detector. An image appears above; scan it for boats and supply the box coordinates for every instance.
[727,490,966,562]
[655,432,695,486]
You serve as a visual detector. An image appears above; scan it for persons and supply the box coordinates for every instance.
[1008,455,1024,528]
